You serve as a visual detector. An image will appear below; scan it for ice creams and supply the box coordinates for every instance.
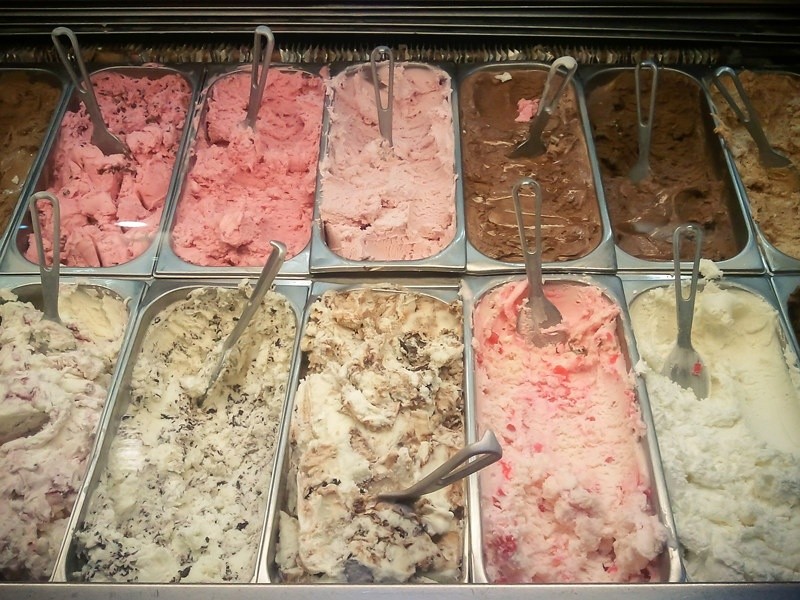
[1,60,800,584]
